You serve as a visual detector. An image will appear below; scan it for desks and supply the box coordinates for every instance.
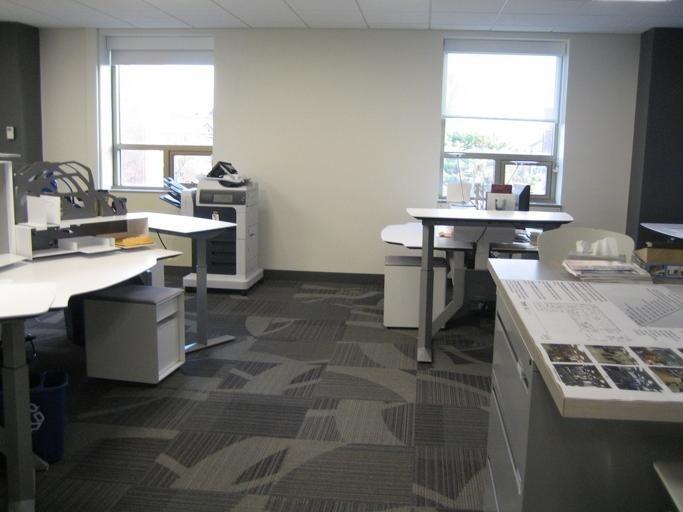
[0,210,238,512]
[486,258,683,512]
[381,207,576,365]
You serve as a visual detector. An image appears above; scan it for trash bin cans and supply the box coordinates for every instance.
[28,368,69,464]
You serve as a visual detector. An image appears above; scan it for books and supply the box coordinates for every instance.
[562,259,653,282]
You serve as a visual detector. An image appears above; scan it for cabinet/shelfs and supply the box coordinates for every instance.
[84,285,186,385]
[383,256,448,329]
